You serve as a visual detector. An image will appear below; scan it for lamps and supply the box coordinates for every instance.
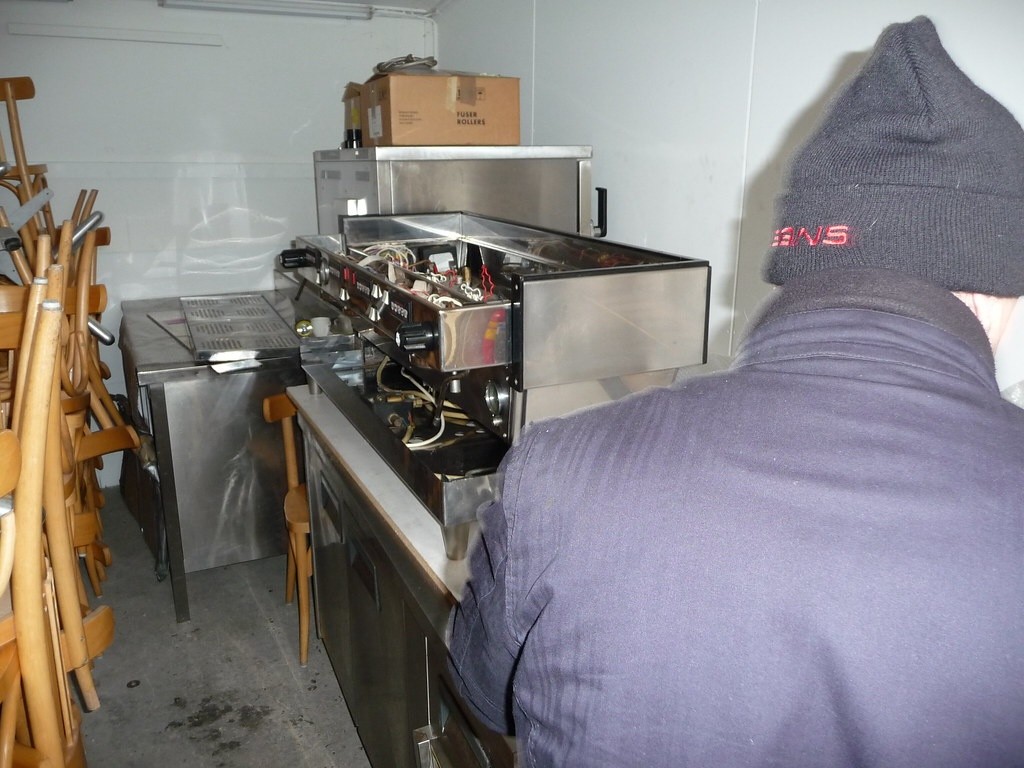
[157,0,376,20]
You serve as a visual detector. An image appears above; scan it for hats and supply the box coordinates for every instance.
[763,16,1024,300]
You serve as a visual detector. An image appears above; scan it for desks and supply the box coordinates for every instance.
[120,288,342,626]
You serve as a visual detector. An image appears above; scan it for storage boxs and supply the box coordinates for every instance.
[359,72,521,145]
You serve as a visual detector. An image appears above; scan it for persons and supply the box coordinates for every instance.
[438,14,1024,768]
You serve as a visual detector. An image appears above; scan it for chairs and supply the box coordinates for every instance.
[260,394,312,663]
[0,77,140,768]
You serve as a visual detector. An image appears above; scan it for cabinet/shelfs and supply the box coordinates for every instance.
[283,384,522,767]
